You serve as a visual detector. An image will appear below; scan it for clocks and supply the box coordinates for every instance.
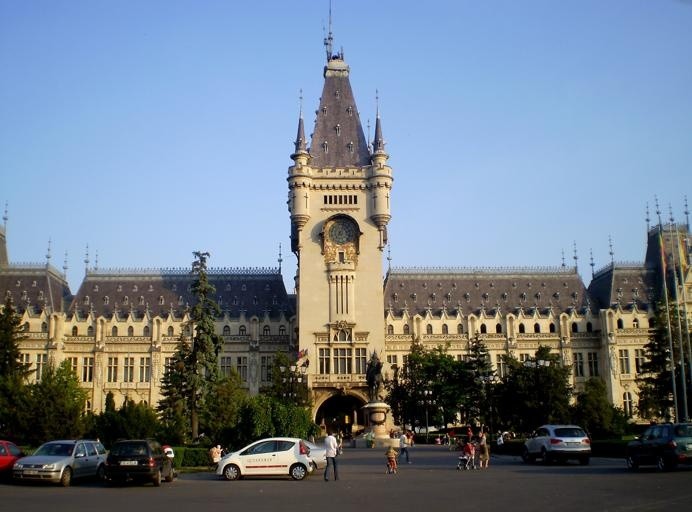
[329,220,354,245]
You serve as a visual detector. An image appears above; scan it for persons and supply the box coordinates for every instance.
[398,428,413,465]
[384,445,399,473]
[331,429,342,455]
[389,428,416,447]
[159,443,178,477]
[208,443,222,472]
[324,429,343,482]
[309,432,315,444]
[338,429,377,448]
[217,444,227,457]
[436,424,538,471]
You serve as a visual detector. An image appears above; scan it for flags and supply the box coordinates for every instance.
[658,227,691,283]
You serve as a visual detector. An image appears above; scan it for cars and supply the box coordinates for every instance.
[522,426,592,464]
[0,439,173,487]
[214,438,327,481]
[626,422,692,471]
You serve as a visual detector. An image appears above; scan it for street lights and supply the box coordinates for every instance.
[279,363,303,413]
[417,386,434,446]
[522,356,556,426]
[478,372,501,445]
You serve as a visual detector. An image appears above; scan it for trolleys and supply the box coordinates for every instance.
[456,443,476,471]
